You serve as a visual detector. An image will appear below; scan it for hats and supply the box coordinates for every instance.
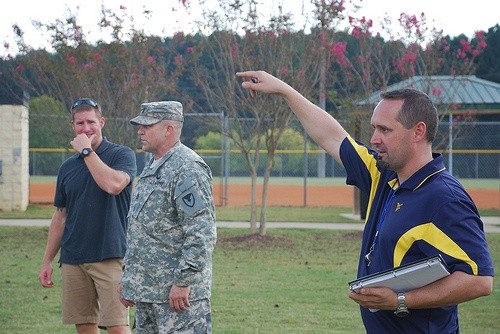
[130,101,184,126]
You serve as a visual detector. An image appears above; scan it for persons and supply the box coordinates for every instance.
[115,101,217,334]
[40,98,138,334]
[235,70,494,334]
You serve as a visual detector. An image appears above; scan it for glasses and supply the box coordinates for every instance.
[71,99,98,109]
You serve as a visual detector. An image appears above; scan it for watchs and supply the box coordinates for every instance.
[79,147,93,159]
[393,293,409,318]
